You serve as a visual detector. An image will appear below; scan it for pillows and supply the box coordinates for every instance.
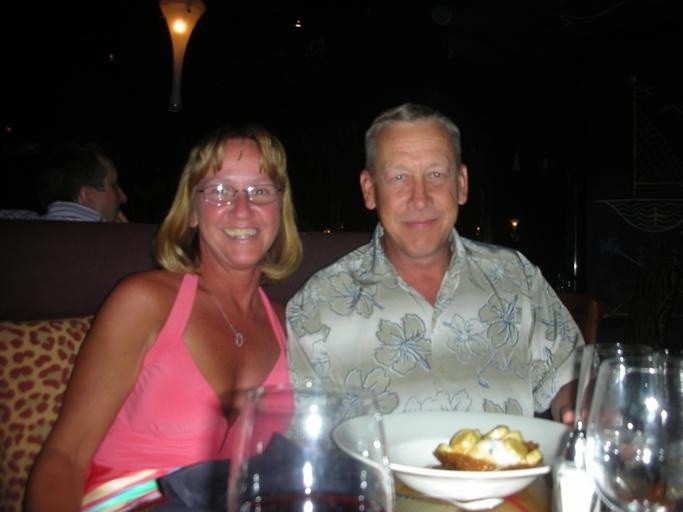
[1,315,94,512]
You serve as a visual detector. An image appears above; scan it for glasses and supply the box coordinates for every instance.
[192,183,281,206]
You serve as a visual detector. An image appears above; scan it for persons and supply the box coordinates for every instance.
[283,101,638,467]
[23,124,304,512]
[0,138,131,224]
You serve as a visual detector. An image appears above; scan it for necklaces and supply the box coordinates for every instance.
[199,276,255,348]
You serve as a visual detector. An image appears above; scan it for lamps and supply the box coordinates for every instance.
[160,1,204,109]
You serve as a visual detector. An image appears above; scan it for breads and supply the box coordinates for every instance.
[433,424,542,469]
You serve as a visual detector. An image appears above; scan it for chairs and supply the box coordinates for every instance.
[559,294,601,346]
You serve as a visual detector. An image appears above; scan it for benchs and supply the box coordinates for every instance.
[0,219,372,321]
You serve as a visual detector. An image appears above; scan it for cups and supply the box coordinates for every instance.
[575,341,683,512]
[223,386,398,512]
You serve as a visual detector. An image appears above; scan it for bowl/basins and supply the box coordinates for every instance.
[330,411,577,503]
